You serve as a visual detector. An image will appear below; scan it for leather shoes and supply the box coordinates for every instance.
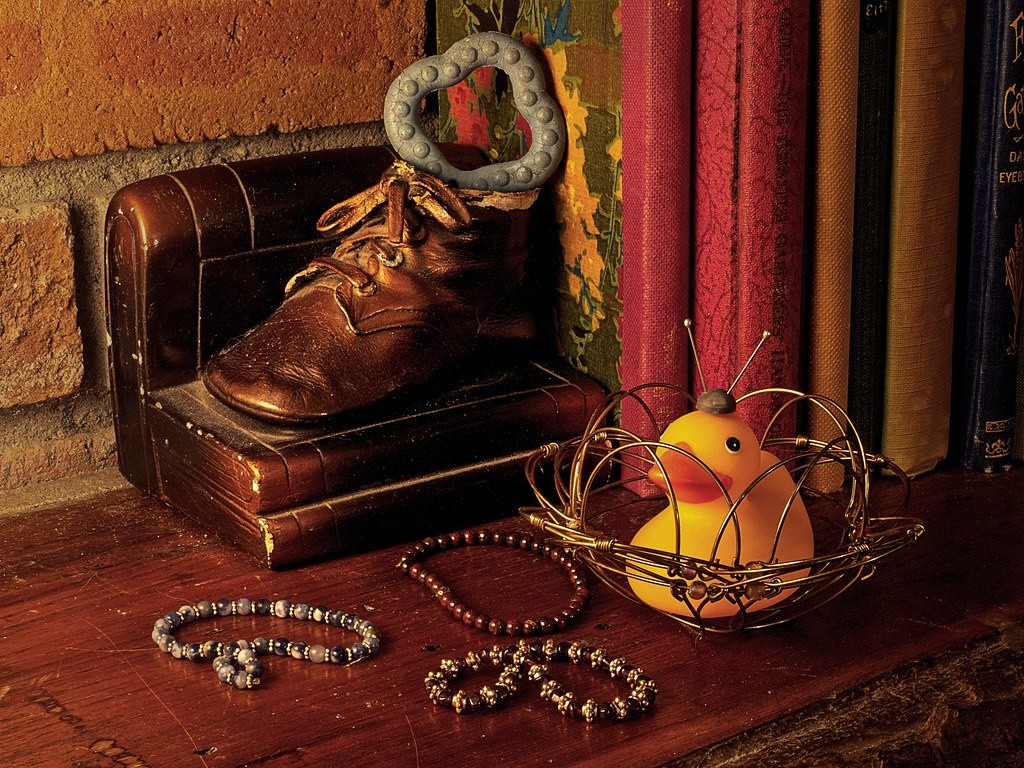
[203,157,544,424]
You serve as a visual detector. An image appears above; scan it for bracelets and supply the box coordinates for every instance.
[424,640,658,724]
[153,596,384,689]
[398,529,590,634]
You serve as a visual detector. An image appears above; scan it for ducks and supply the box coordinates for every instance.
[625,411,813,618]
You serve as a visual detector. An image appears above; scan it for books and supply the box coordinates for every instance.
[425,1,1024,503]
